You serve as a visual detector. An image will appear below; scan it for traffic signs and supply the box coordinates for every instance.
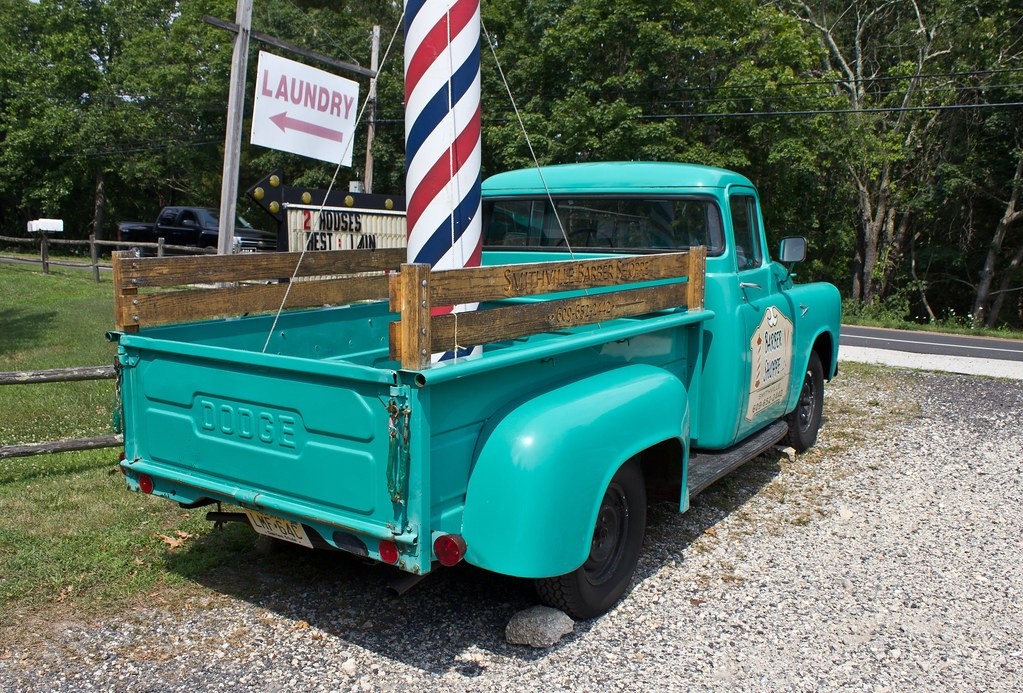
[250,50,361,168]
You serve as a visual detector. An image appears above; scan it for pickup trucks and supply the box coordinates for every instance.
[117,205,279,257]
[104,156,844,623]
